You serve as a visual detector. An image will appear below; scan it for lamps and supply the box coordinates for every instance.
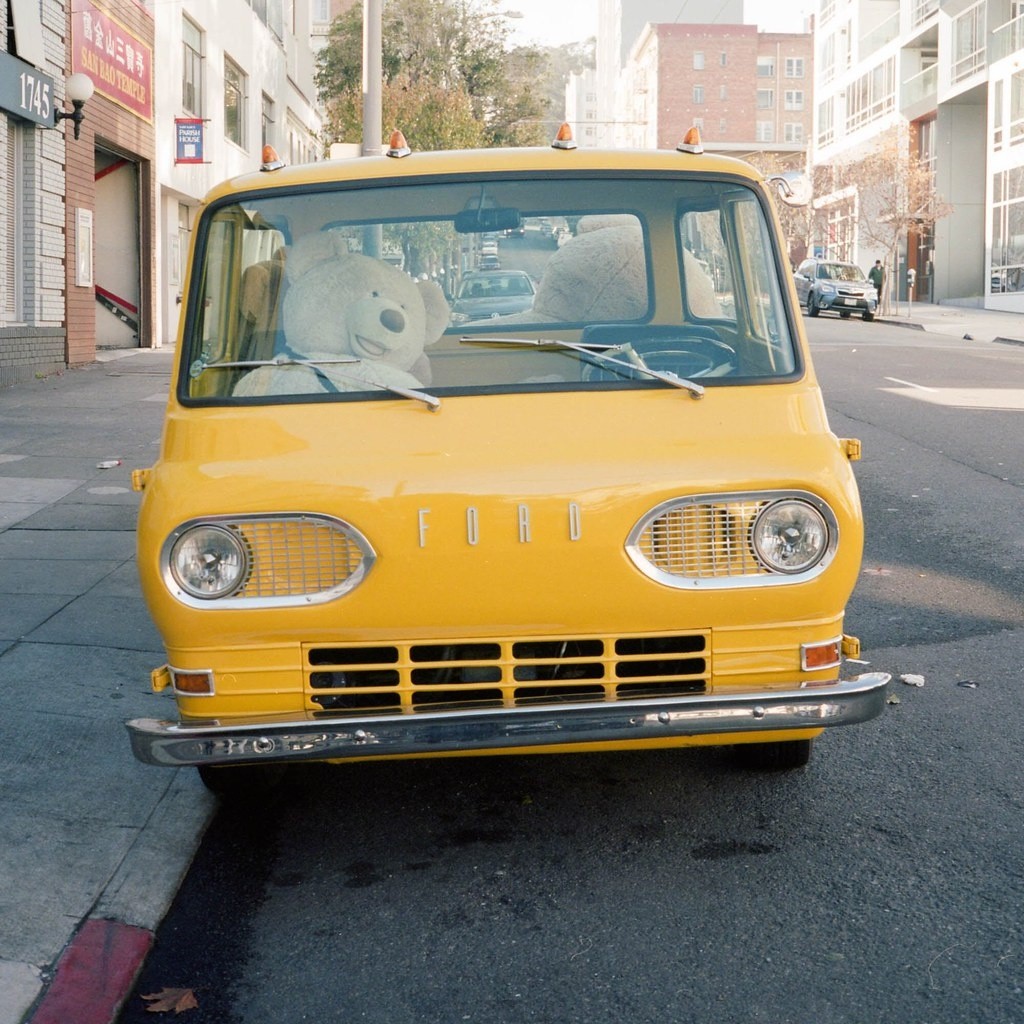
[53,73,95,141]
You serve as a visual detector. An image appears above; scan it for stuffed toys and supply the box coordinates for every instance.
[457,213,725,329]
[233,229,450,398]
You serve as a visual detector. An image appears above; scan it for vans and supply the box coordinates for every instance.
[383,254,405,270]
[126,129,892,808]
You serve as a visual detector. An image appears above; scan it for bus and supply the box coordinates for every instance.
[506,217,525,238]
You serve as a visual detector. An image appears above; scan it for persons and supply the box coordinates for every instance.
[869,260,886,305]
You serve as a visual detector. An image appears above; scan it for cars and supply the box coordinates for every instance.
[539,220,573,248]
[479,231,500,270]
[451,270,538,326]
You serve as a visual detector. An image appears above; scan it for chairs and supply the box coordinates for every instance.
[582,324,728,381]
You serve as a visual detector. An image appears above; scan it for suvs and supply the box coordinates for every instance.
[792,256,879,321]
